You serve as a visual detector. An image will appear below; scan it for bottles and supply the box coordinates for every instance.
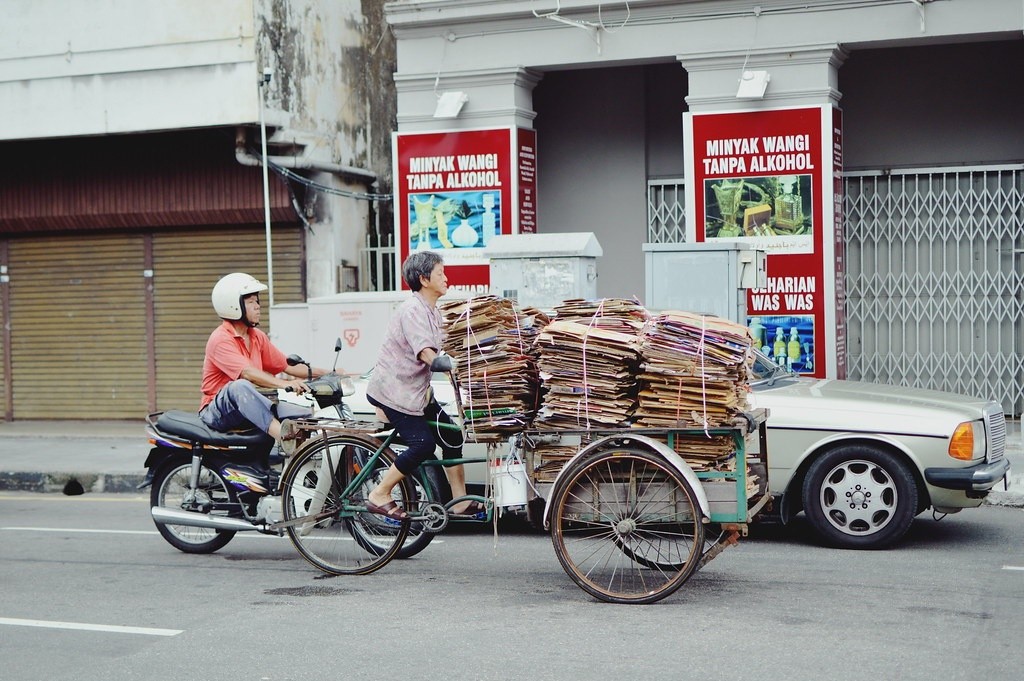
[773,327,786,356]
[749,318,767,346]
[787,327,801,363]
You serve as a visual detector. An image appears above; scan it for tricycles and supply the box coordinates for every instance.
[268,364,777,604]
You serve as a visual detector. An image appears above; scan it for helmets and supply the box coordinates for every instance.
[211,273,268,320]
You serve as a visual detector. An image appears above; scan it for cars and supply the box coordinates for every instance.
[282,344,1012,551]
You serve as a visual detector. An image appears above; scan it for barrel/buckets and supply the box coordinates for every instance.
[489,464,527,506]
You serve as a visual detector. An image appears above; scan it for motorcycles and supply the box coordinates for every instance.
[136,336,450,554]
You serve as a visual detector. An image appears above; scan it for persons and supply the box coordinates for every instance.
[367,251,486,520]
[198,272,344,456]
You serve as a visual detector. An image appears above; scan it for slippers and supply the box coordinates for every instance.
[454,500,486,516]
[367,499,411,520]
